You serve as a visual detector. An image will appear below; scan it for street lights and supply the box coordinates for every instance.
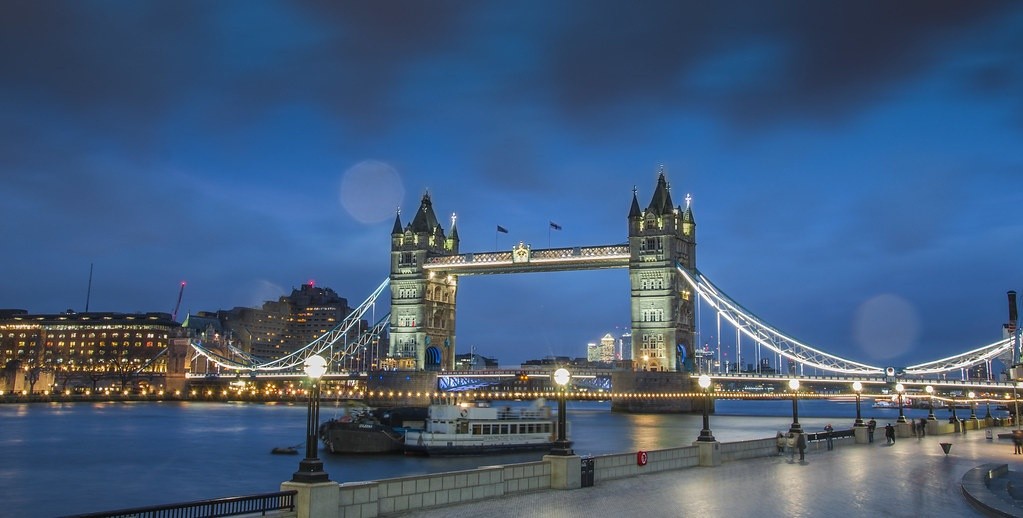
[696,374,716,441]
[968,392,977,419]
[926,386,936,420]
[896,384,906,422]
[985,392,992,418]
[949,392,958,423]
[293,355,329,482]
[853,382,866,427]
[549,369,574,455]
[789,378,802,434]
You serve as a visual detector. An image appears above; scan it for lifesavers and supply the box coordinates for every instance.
[637,451,648,466]
[460,410,468,418]
[344,416,347,421]
[347,417,353,422]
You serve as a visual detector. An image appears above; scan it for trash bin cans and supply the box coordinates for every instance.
[1003,419,1011,427]
[581,458,595,487]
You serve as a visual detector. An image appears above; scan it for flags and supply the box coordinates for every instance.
[549,220,563,230]
[497,224,508,234]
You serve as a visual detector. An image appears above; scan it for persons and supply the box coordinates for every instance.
[788,433,796,458]
[798,434,807,460]
[824,424,834,451]
[886,423,896,444]
[911,418,917,436]
[920,417,928,436]
[776,431,784,455]
[867,417,876,443]
[1011,430,1023,453]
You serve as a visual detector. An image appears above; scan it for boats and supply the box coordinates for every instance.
[403,397,572,455]
[872,399,908,408]
[321,398,428,454]
